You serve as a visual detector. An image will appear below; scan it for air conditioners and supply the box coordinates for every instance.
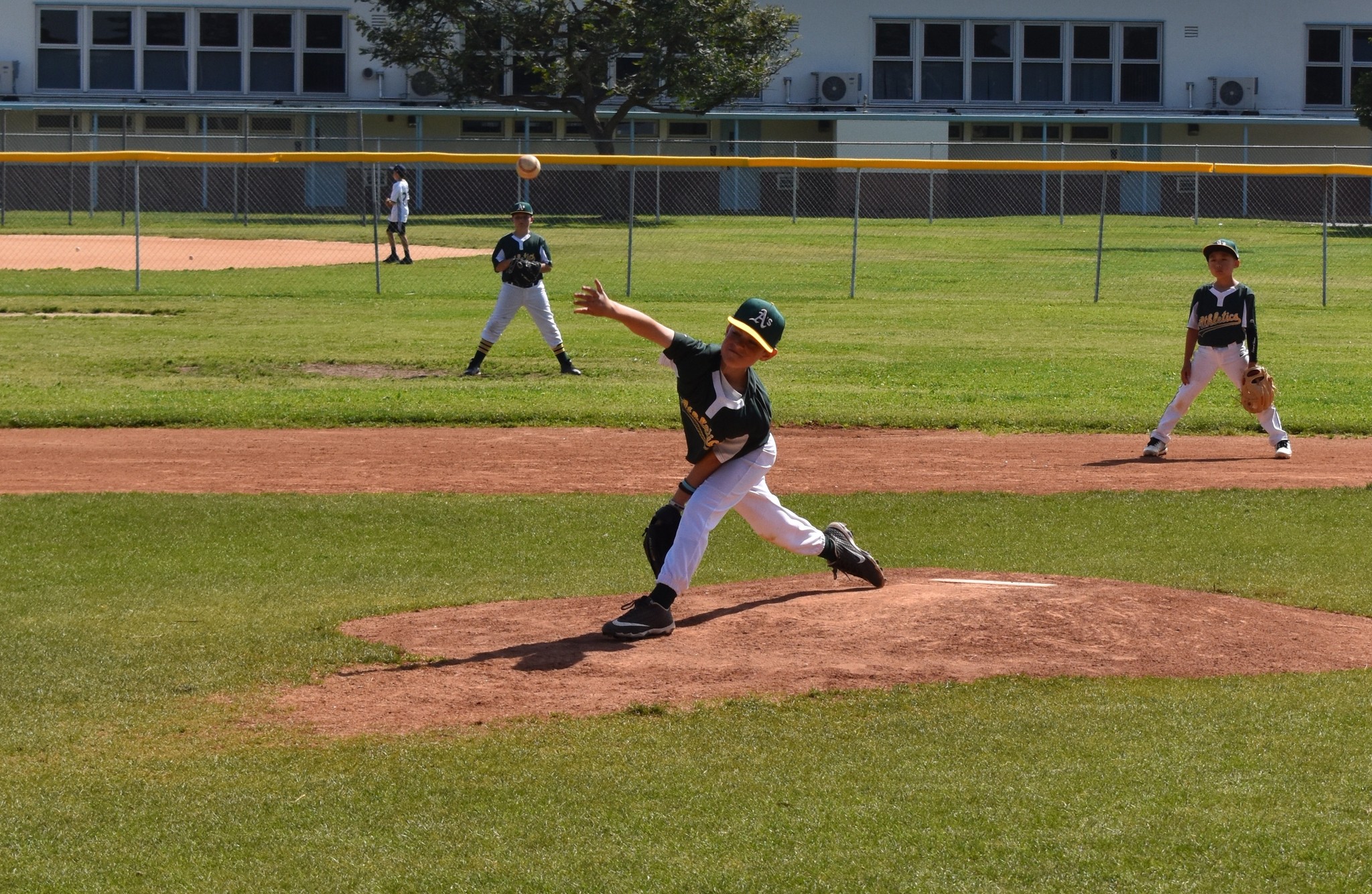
[811,72,859,106]
[407,60,463,100]
[0,61,14,94]
[1207,76,1255,110]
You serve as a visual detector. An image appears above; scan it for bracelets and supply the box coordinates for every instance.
[678,478,695,495]
[669,499,685,510]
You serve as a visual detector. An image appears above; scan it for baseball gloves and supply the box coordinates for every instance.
[385,197,393,209]
[642,503,681,580]
[1242,366,1277,414]
[506,257,542,287]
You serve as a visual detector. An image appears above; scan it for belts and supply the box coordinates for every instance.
[505,279,538,288]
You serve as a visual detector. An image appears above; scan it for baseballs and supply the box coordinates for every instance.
[516,154,541,179]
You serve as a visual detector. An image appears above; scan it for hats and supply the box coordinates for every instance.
[1203,239,1240,261]
[510,202,533,217]
[389,164,407,176]
[727,298,786,353]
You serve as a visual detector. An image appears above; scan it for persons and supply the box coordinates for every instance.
[466,202,581,375]
[573,278,886,638]
[383,164,412,265]
[1143,239,1293,458]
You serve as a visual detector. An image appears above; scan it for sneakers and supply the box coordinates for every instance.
[601,595,677,640]
[559,361,582,376]
[465,358,482,376]
[1143,437,1167,456]
[823,522,887,589]
[1275,438,1292,459]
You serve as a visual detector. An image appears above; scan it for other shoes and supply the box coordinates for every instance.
[399,256,412,264]
[383,255,399,263]
